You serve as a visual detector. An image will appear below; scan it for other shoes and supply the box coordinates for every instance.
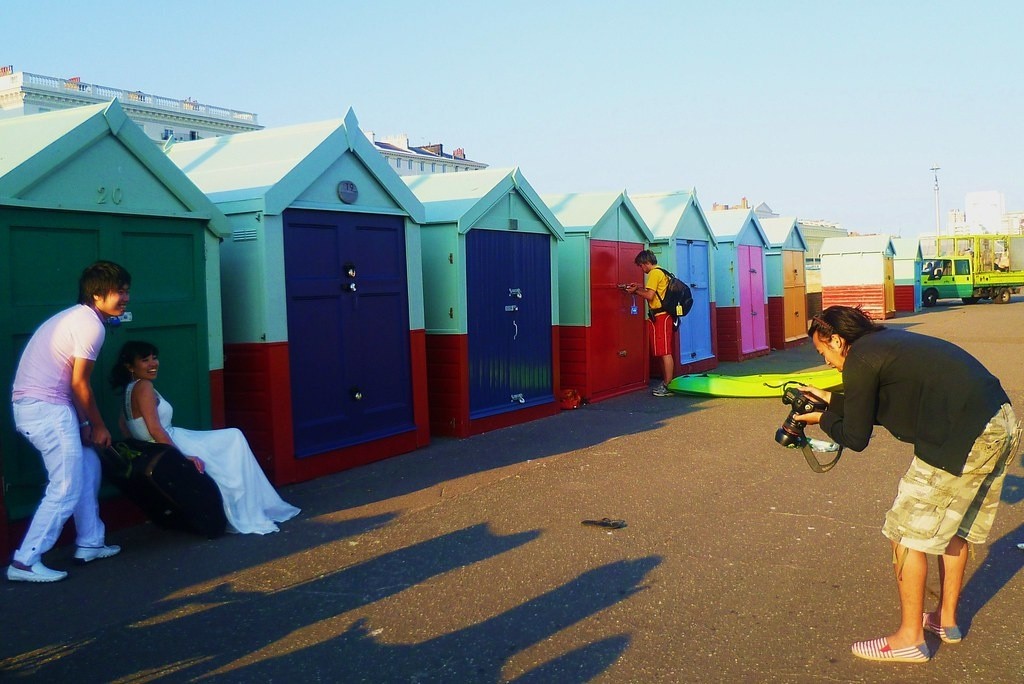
[852,635,930,663]
[7,563,68,584]
[74,544,120,564]
[923,612,961,641]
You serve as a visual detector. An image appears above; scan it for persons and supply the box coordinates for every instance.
[793,305,1022,663]
[626,250,675,397]
[110,341,301,535]
[7,261,131,582]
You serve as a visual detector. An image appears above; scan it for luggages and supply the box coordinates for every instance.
[100,438,230,535]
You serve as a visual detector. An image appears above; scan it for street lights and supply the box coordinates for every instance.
[931,162,941,237]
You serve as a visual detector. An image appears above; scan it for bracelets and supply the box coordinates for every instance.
[80,421,89,427]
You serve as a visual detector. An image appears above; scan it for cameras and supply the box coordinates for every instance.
[774,387,828,449]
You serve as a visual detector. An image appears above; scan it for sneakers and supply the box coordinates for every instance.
[652,382,674,396]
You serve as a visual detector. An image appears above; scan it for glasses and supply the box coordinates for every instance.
[811,315,834,330]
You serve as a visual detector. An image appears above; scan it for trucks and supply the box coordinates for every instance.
[919,234,1024,307]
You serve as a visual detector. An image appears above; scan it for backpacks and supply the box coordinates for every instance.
[648,268,693,317]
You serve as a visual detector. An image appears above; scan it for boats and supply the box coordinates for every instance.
[667,370,848,397]
